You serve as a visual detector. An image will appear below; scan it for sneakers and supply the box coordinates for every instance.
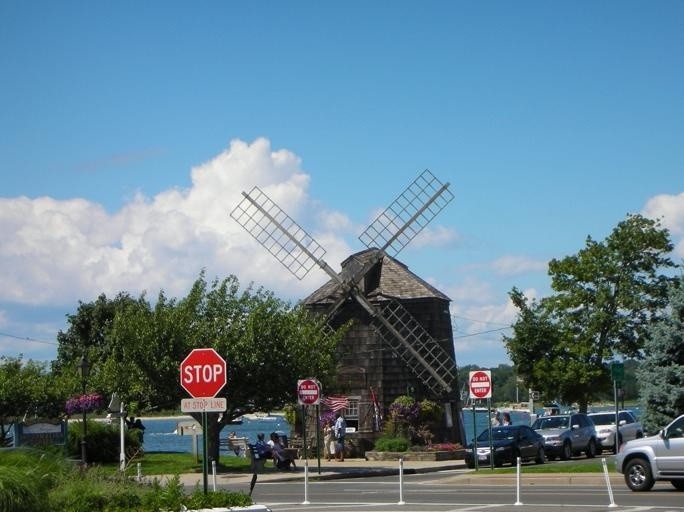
[327,457,344,462]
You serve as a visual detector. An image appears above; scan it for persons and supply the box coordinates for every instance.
[134,418,145,448]
[323,418,334,462]
[333,411,346,462]
[489,410,503,426]
[267,430,300,471]
[124,414,135,430]
[255,430,289,471]
[501,412,512,427]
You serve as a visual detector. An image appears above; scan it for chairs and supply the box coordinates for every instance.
[247,443,272,460]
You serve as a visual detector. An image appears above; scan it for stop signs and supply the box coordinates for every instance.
[297,379,322,405]
[179,348,227,398]
[469,371,492,398]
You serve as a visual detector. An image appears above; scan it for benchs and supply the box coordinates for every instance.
[287,437,313,451]
[219,437,249,457]
[269,447,300,468]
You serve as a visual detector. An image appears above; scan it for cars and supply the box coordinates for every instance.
[464,411,644,468]
[613,414,684,492]
